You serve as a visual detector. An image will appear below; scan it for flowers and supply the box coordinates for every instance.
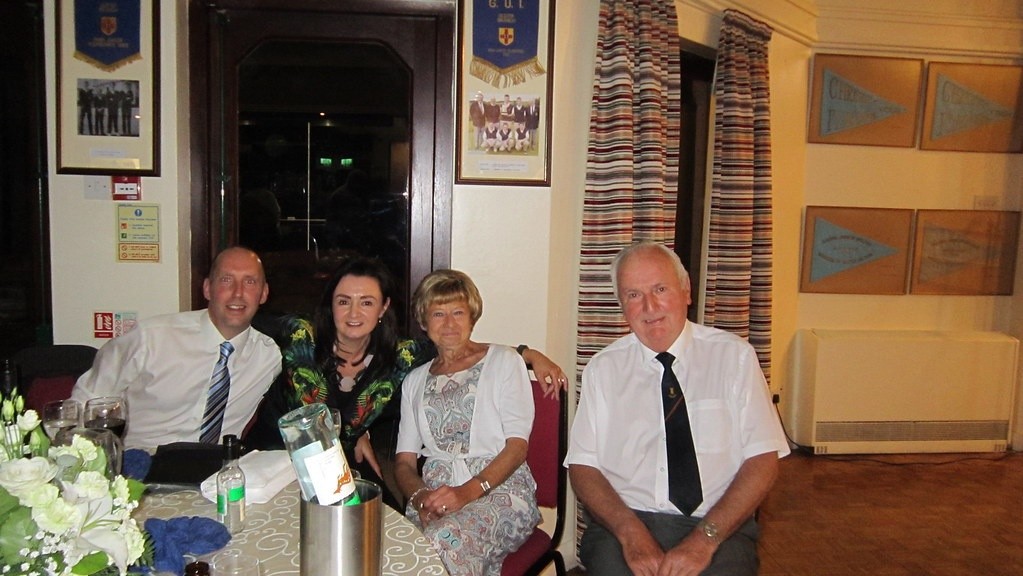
[1,387,157,576]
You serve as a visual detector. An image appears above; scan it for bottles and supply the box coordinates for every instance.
[217,434,246,534]
[185,561,210,576]
[277,402,362,505]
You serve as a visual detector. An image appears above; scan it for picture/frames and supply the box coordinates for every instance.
[455,0,555,187]
[54,0,161,177]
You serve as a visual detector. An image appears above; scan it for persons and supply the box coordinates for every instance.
[61,248,383,486]
[285,260,569,514]
[393,269,544,576]
[469,92,539,154]
[77,81,135,136]
[563,241,790,576]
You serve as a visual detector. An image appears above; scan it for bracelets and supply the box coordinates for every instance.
[366,429,370,440]
[409,487,429,506]
[516,345,533,371]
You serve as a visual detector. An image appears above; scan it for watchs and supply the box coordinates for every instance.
[474,474,491,495]
[696,519,722,544]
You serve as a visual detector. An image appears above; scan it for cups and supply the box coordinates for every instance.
[299,479,384,576]
[328,408,341,438]
[42,396,128,483]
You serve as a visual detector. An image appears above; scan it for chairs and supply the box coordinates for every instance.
[500,364,569,576]
[5,345,98,424]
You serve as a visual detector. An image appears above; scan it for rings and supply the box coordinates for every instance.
[427,512,432,515]
[442,505,447,511]
[558,378,565,382]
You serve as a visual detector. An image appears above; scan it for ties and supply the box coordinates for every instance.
[200,341,234,443]
[656,352,704,518]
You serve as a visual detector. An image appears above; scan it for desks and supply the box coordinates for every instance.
[132,484,450,576]
